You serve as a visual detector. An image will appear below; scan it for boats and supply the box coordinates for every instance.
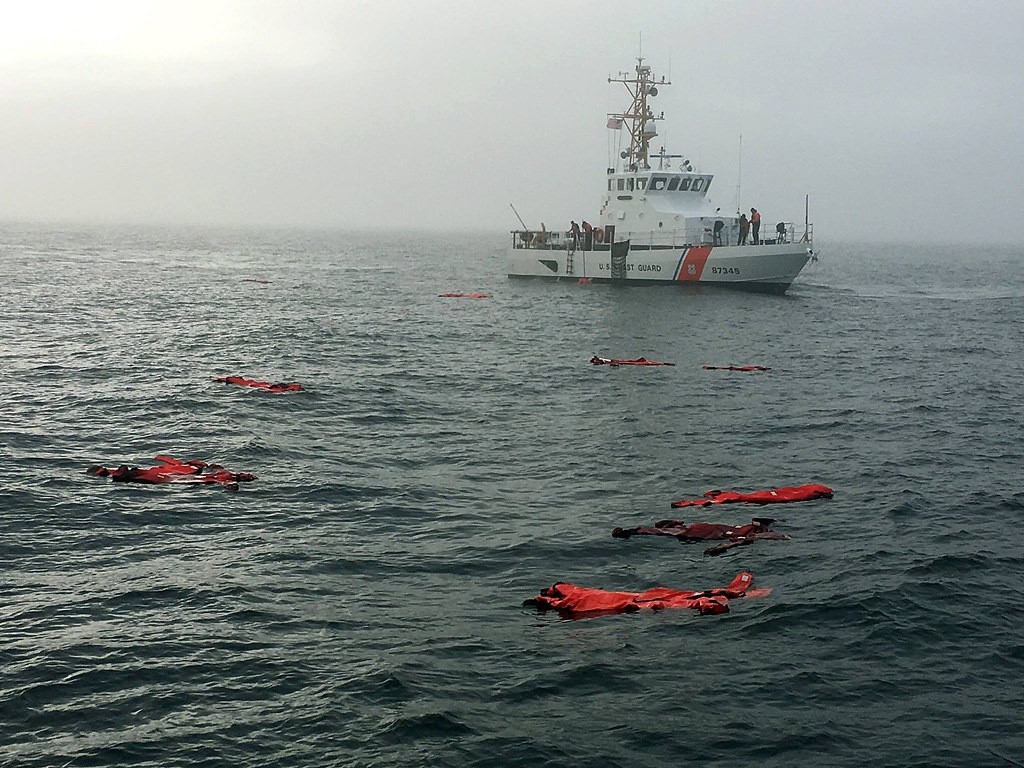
[505,32,816,295]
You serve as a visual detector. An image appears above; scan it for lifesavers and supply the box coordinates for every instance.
[594,228,605,242]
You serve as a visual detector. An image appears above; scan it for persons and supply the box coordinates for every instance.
[738,213,750,245]
[582,221,592,251]
[749,208,761,245]
[567,220,580,251]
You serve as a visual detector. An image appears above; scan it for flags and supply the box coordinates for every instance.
[606,119,623,130]
